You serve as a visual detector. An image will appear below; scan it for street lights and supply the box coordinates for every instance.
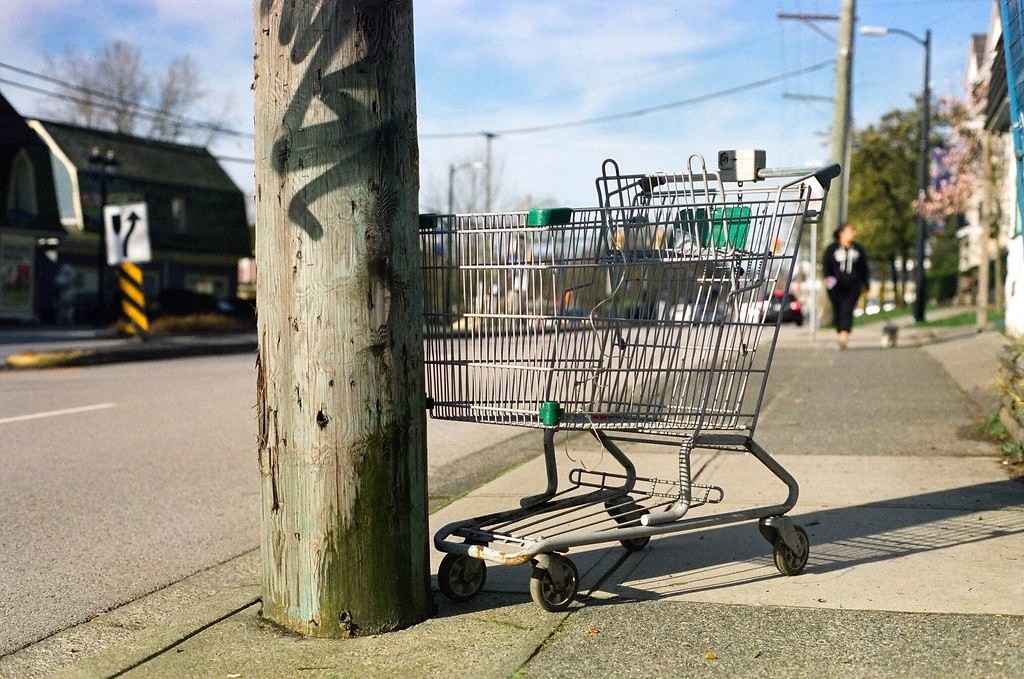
[860,25,931,322]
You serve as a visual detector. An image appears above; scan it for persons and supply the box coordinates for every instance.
[54,265,78,327]
[822,224,869,350]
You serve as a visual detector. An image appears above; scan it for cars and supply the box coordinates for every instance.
[669,302,726,325]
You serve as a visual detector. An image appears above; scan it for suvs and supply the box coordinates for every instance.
[760,292,802,326]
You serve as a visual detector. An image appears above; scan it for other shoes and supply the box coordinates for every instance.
[837,339,846,349]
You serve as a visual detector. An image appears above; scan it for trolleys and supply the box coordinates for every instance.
[415,148,842,613]
